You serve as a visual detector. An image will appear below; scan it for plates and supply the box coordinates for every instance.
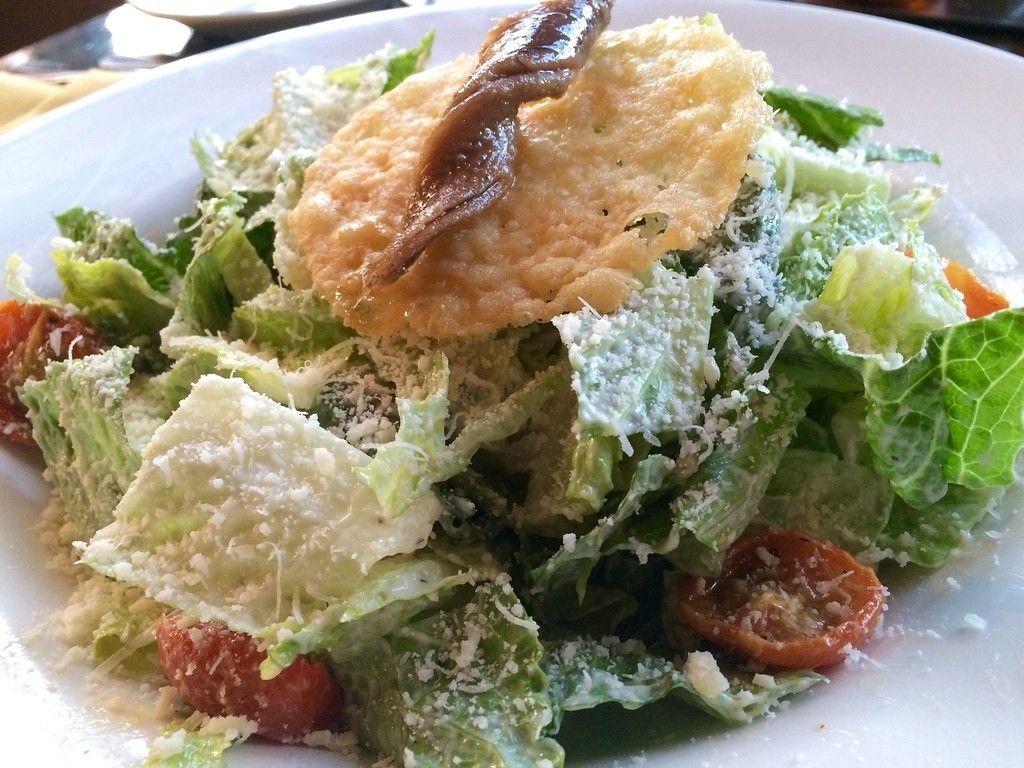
[128,0,366,36]
[0,0,1024,768]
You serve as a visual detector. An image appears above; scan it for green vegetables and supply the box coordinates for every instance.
[23,35,1024,768]
